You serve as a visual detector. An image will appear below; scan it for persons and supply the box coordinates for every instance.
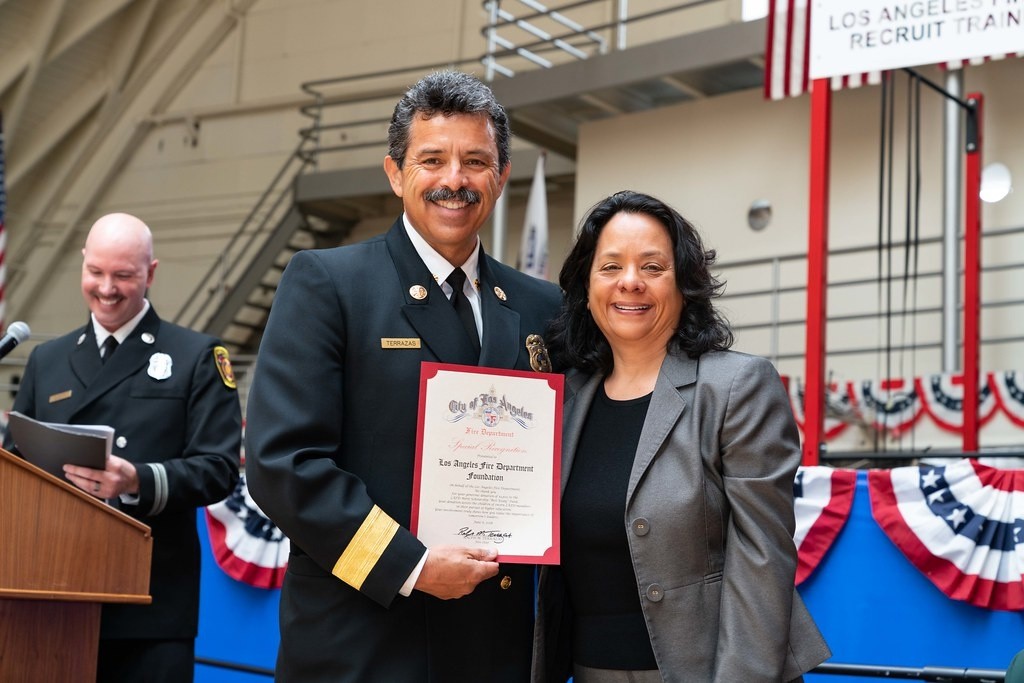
[246,72,566,683]
[525,188,832,683]
[2,213,243,683]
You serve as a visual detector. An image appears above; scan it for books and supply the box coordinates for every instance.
[5,410,115,505]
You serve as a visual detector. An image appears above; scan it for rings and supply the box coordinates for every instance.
[93,481,101,491]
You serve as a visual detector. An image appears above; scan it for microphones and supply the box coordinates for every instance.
[0,321,31,360]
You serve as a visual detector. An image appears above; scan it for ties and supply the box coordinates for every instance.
[101,336,119,364]
[444,268,482,358]
